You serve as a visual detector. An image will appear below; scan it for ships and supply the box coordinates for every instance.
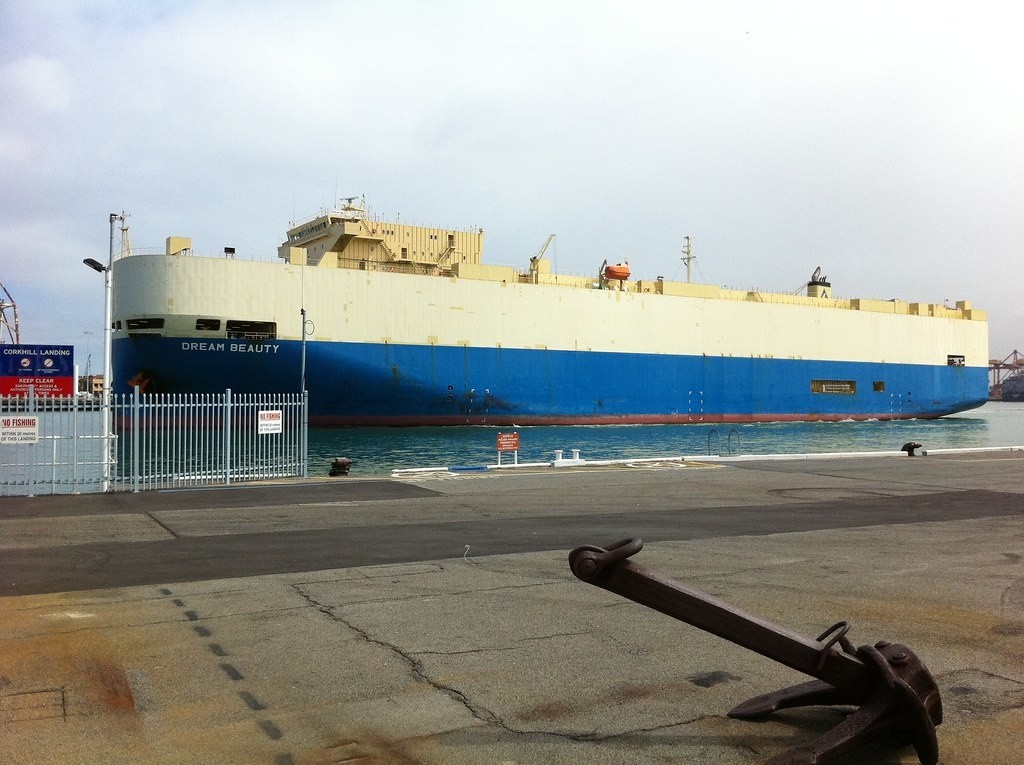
[105,193,991,433]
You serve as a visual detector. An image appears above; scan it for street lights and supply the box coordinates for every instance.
[82,257,115,493]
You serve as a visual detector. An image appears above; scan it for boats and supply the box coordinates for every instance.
[1001,375,1024,402]
